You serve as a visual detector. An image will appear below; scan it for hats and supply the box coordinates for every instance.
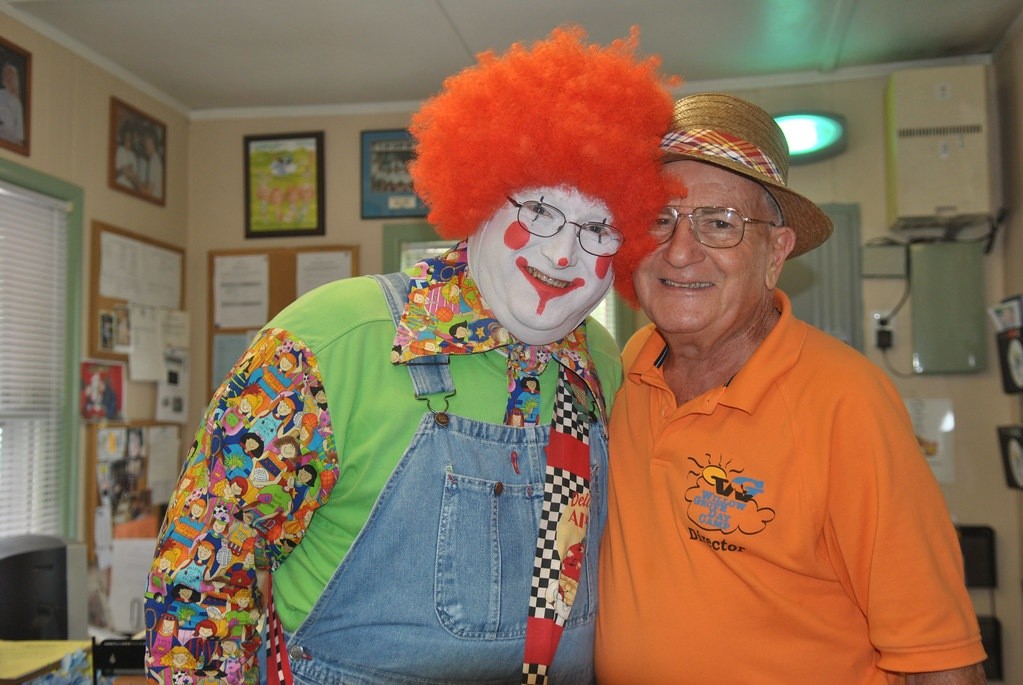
[654,93,834,260]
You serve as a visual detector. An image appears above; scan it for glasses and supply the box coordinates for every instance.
[504,195,624,258]
[651,206,774,249]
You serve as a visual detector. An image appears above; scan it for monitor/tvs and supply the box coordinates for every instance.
[0,533,89,640]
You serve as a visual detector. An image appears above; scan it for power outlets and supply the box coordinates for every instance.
[869,309,898,350]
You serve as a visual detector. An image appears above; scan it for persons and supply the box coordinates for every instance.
[142,36,680,685]
[0,63,24,145]
[84,373,118,417]
[115,128,163,198]
[598,96,990,685]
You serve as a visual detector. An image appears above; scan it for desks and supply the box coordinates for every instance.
[0,640,92,685]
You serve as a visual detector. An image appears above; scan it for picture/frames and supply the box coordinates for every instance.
[359,128,429,220]
[242,130,327,237]
[0,36,33,156]
[110,96,167,204]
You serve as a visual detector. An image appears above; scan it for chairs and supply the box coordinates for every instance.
[90,634,148,685]
